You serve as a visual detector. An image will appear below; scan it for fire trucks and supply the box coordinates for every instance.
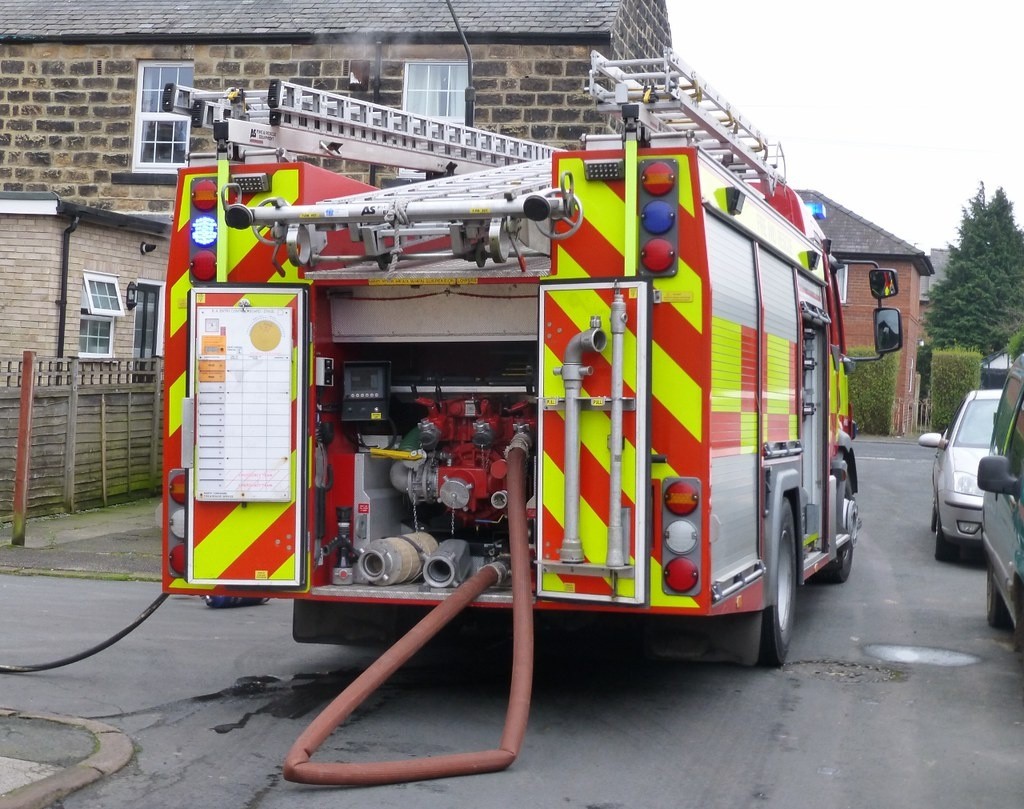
[148,48,905,674]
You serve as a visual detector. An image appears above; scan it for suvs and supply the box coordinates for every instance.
[975,352,1024,653]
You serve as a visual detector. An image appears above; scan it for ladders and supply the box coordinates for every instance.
[589,47,787,197]
[162,78,568,175]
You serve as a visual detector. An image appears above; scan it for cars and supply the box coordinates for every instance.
[917,388,1003,561]
